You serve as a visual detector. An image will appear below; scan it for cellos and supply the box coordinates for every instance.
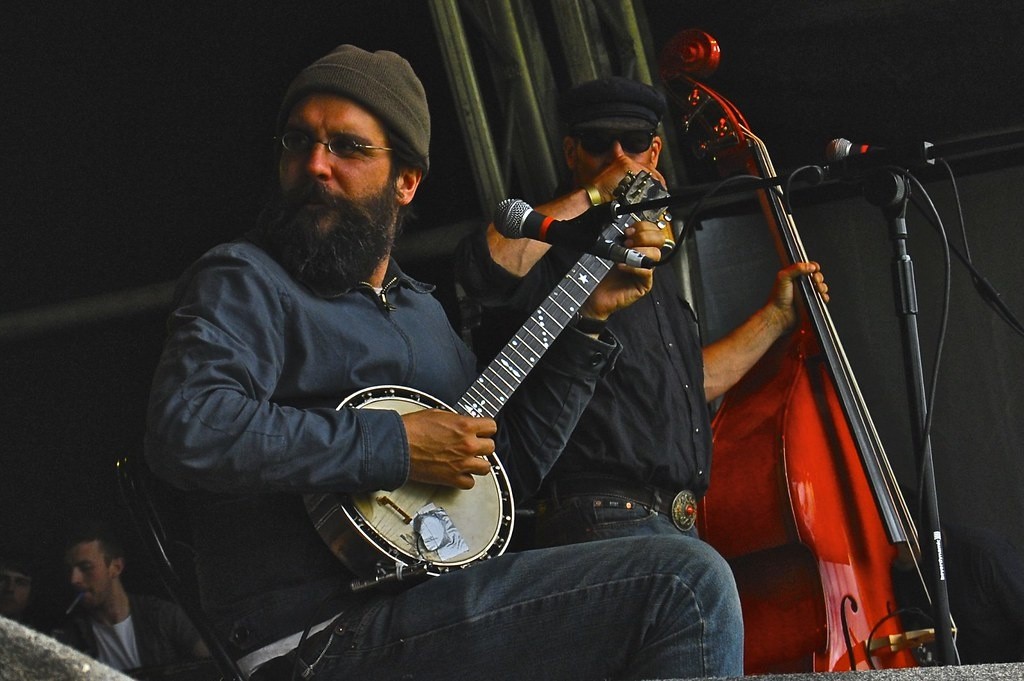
[656,27,961,673]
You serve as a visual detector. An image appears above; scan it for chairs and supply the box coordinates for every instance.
[115,438,249,681]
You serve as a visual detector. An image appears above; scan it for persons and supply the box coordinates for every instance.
[50,526,211,671]
[0,554,36,621]
[144,45,744,681]
[889,483,1024,667]
[436,77,831,550]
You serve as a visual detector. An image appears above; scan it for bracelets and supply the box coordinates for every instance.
[583,182,601,206]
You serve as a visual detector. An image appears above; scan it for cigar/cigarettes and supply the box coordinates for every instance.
[66,592,82,614]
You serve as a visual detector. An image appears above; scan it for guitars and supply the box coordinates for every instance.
[302,169,674,591]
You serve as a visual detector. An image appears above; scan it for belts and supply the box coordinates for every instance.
[534,477,698,532]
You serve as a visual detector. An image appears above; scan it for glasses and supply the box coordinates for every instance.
[272,132,394,156]
[570,130,654,154]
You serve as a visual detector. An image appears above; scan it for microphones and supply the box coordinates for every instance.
[826,138,938,168]
[493,198,655,270]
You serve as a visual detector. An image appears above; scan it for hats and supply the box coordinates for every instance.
[276,44,430,184]
[566,76,665,129]
